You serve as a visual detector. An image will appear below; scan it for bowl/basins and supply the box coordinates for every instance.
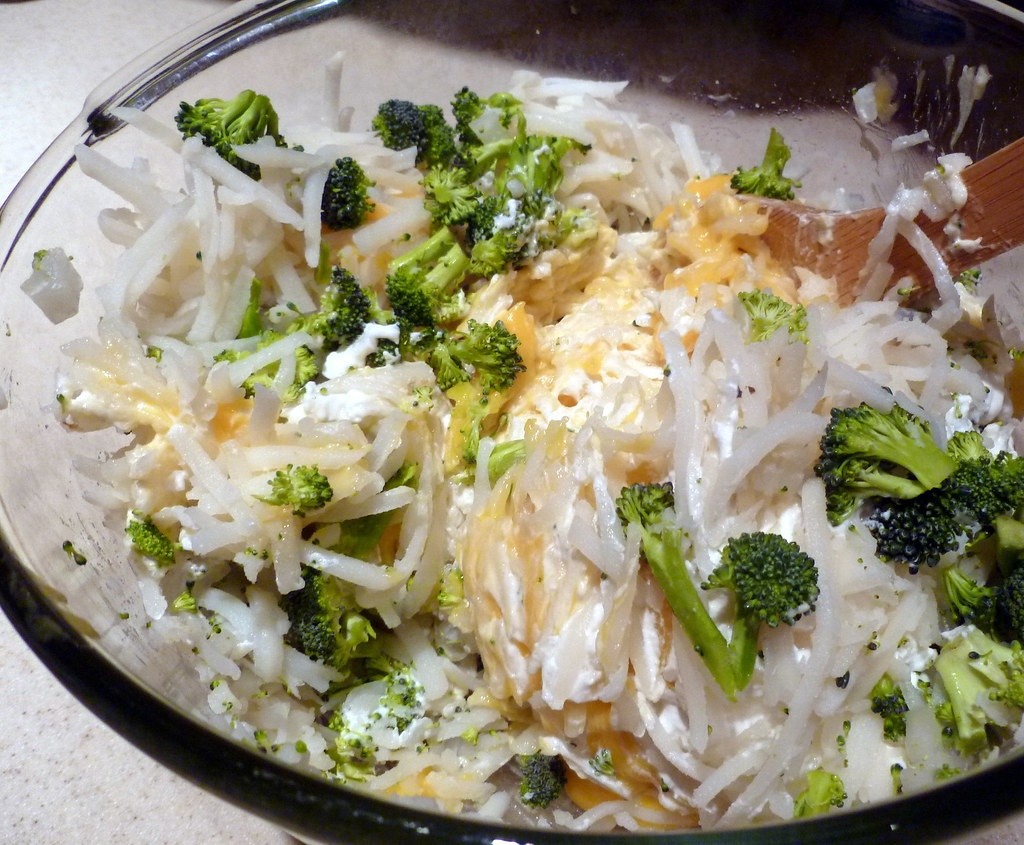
[0,0,1024,845]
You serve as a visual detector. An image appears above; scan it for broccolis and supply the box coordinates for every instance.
[120,87,1024,819]
